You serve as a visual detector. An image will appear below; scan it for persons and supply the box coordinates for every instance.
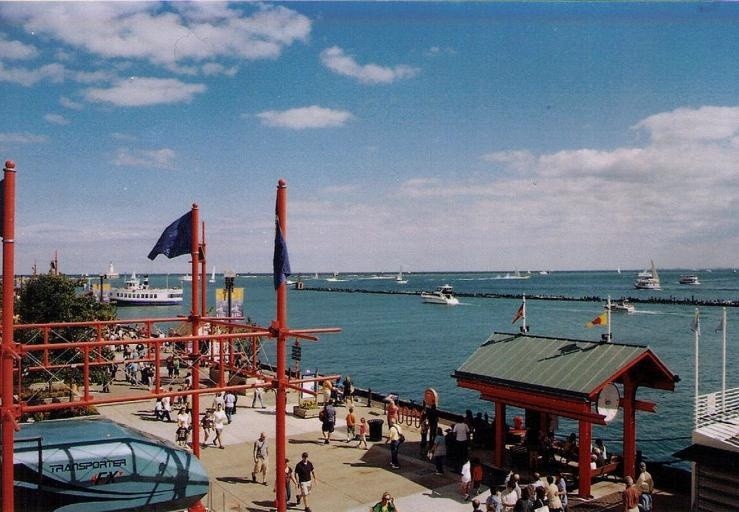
[105,323,402,512]
[420,404,655,512]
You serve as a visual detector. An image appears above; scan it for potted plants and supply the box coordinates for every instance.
[292,399,325,418]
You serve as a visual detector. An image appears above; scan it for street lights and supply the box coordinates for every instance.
[99,271,106,303]
[222,266,238,362]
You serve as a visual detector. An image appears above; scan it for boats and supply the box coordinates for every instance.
[178,272,201,282]
[677,273,703,287]
[539,271,550,275]
[600,298,635,315]
[109,270,185,306]
[285,269,393,286]
[419,283,457,305]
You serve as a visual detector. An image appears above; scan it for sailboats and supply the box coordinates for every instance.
[632,255,667,291]
[508,263,531,280]
[208,265,216,283]
[616,266,623,274]
[106,262,120,279]
[394,263,408,286]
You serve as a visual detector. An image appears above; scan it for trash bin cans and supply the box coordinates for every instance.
[367,419,384,441]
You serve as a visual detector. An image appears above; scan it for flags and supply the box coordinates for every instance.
[148,210,193,261]
[273,196,291,291]
[586,312,608,328]
[512,303,523,325]
